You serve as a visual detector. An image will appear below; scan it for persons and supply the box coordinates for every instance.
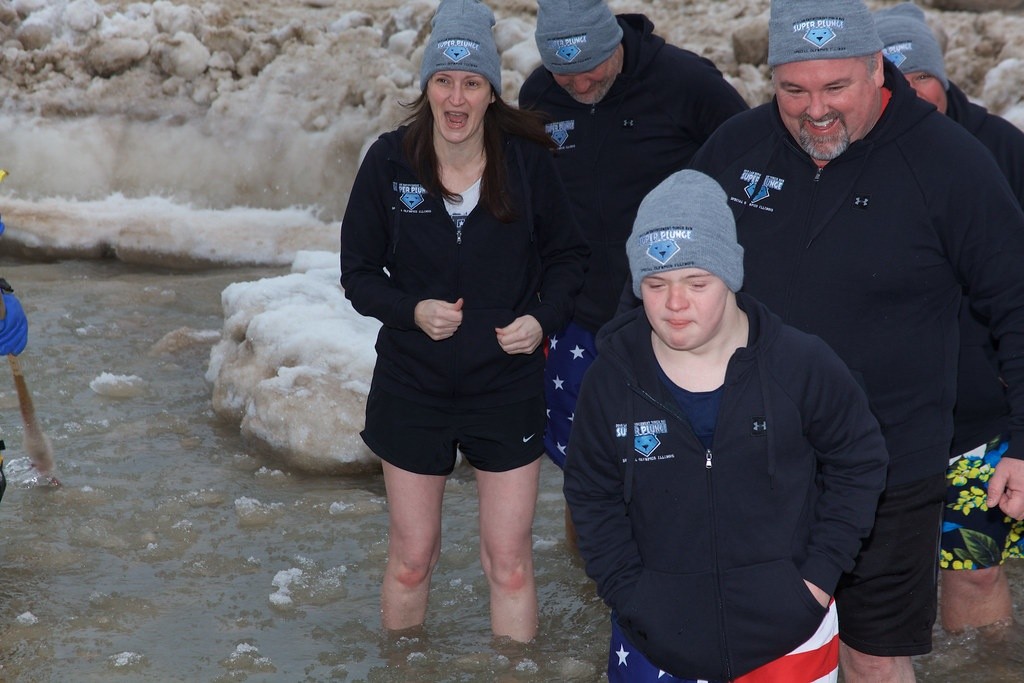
[515,1,759,566]
[870,1,1024,638]
[561,167,888,682]
[341,0,597,644]
[676,1,1024,683]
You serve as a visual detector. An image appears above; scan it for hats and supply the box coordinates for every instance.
[768,1,883,68]
[419,0,502,93]
[535,0,624,72]
[625,169,745,301]
[873,3,950,91]
